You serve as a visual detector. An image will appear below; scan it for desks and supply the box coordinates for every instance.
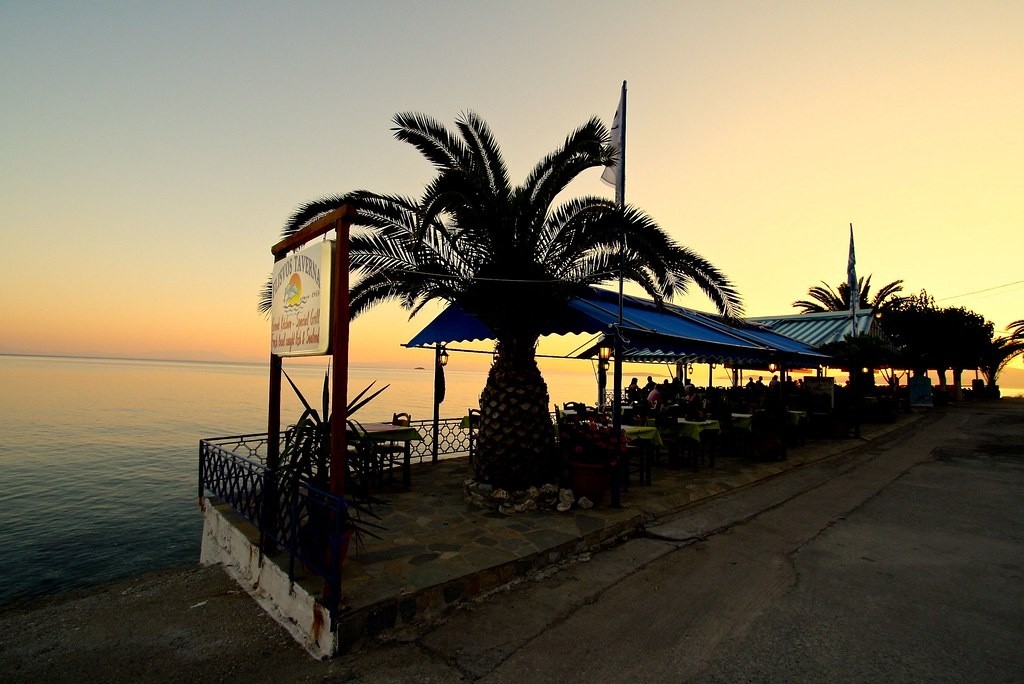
[731,412,760,460]
[789,411,807,449]
[560,410,593,432]
[347,427,423,487]
[650,417,720,473]
[553,423,663,486]
[621,406,634,416]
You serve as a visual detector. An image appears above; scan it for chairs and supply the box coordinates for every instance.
[347,383,947,484]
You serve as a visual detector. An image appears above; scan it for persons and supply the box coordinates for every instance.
[629,375,804,416]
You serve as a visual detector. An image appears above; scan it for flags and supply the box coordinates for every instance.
[848,229,860,314]
[601,86,627,203]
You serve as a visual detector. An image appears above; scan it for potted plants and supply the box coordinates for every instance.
[249,367,391,572]
